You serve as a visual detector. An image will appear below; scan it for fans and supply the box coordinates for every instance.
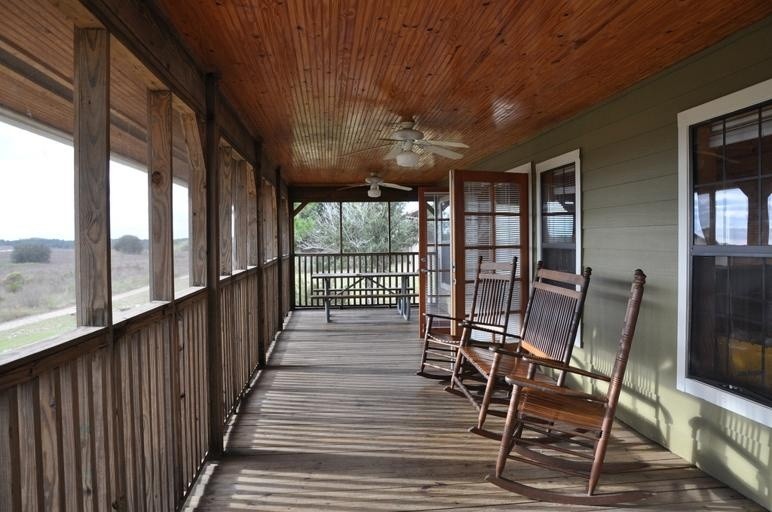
[336,173,412,198]
[376,119,469,168]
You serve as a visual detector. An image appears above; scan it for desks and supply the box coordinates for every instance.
[310,272,419,323]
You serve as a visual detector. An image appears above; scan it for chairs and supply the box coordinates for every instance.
[442,260,595,445]
[419,256,518,388]
[484,269,646,507]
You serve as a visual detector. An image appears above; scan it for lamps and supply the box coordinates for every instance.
[367,184,381,198]
[395,143,418,168]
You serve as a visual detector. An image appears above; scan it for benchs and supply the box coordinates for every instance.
[310,286,419,322]
